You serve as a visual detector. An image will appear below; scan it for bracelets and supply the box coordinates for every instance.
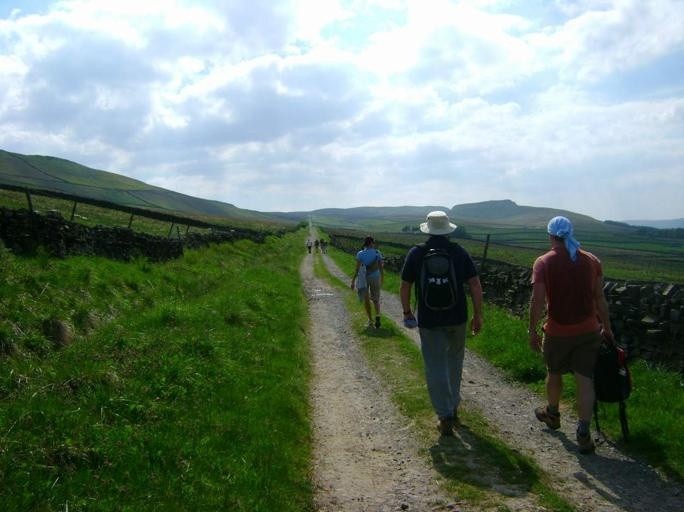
[402,310,412,314]
[528,327,535,335]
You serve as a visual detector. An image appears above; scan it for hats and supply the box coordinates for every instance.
[419,211,457,236]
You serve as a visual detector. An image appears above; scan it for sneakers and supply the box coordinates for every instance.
[575,435,595,454]
[534,405,561,430]
[437,418,452,435]
[364,320,373,327]
[374,316,380,327]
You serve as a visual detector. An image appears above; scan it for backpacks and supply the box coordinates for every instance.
[594,330,631,403]
[415,242,459,311]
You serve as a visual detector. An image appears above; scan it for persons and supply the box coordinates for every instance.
[325,241,327,253]
[306,240,312,253]
[399,211,483,435]
[350,235,383,328]
[320,238,326,254]
[314,239,319,253]
[528,215,614,454]
